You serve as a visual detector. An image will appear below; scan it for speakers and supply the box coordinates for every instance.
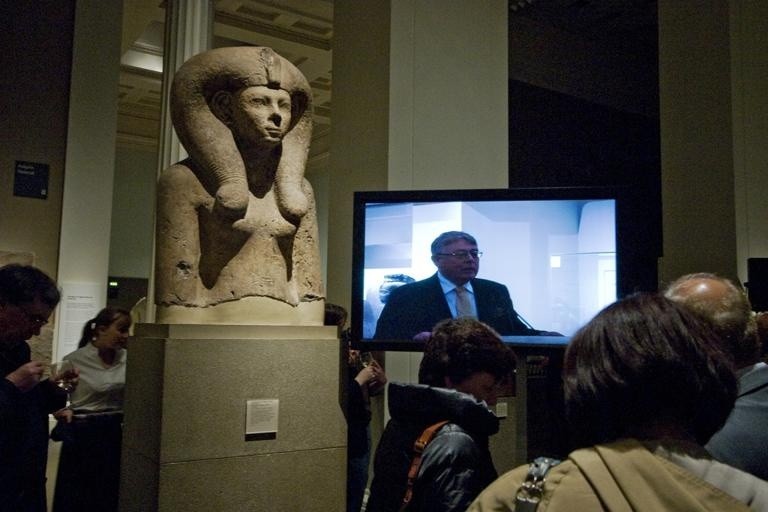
[747,258,768,314]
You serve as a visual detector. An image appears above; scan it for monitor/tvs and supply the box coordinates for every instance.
[350,186,628,356]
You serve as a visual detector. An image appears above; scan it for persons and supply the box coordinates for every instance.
[663,272,768,483]
[324,303,380,511]
[372,231,564,335]
[467,292,768,512]
[48,305,133,511]
[155,46,325,308]
[0,263,80,510]
[365,316,519,512]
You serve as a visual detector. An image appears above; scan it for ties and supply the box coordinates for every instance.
[457,288,472,322]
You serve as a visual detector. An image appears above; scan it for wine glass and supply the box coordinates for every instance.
[48,360,78,410]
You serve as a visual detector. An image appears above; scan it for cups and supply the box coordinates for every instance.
[359,352,373,368]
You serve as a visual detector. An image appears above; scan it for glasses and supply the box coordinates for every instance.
[440,251,484,261]
[21,306,48,325]
